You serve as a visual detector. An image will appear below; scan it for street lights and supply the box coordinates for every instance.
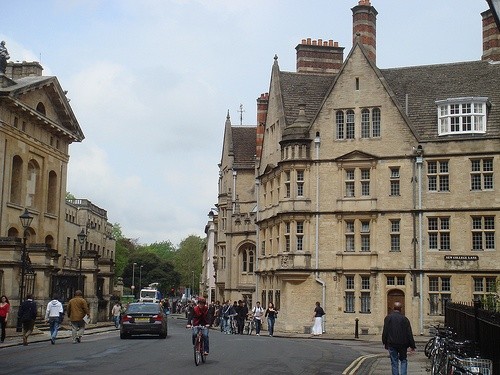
[132,263,137,294]
[192,271,194,295]
[76,228,87,294]
[15,208,34,332]
[140,265,144,291]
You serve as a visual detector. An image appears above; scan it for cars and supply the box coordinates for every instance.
[120,302,168,339]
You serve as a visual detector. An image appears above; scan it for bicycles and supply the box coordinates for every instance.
[425,323,494,375]
[215,313,256,335]
[186,326,208,365]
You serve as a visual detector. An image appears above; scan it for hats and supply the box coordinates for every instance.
[198,297,206,303]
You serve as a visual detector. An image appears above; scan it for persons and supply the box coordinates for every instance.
[111,300,125,328]
[311,302,326,336]
[205,300,214,326]
[44,292,63,344]
[173,301,177,313]
[221,299,233,335]
[0,41,10,73]
[18,295,36,345]
[382,302,417,375]
[235,300,248,336]
[67,290,89,343]
[0,295,10,344]
[264,302,278,337]
[186,296,197,327]
[160,298,170,319]
[215,301,222,327]
[252,301,263,336]
[176,300,183,314]
[230,302,239,334]
[187,297,212,355]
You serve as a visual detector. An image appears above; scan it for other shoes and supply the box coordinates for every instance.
[1,340,4,343]
[76,337,80,343]
[73,342,76,344]
[24,343,28,346]
[50,338,55,344]
[23,336,27,343]
[204,352,208,355]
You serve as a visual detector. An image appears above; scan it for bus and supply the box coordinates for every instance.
[139,289,162,304]
